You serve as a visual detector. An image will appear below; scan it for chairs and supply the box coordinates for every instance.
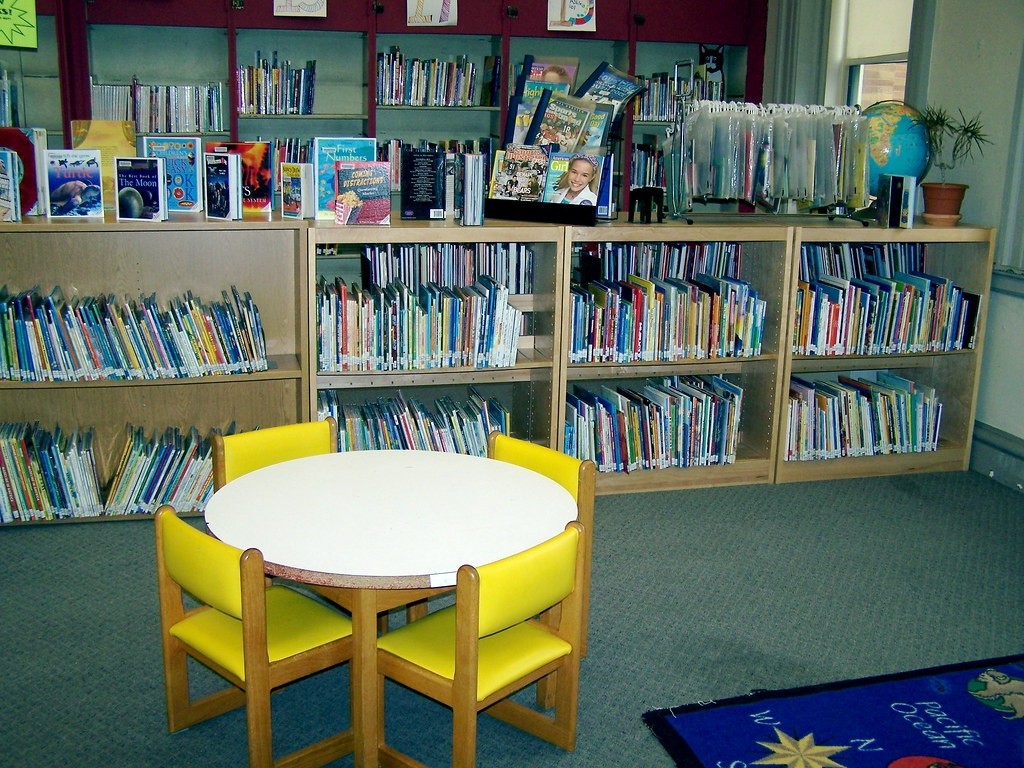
[155,417,597,767]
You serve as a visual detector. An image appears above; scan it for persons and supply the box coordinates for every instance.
[550,153,600,206]
[505,176,518,197]
[507,158,518,175]
[0,127,614,238]
[562,115,575,137]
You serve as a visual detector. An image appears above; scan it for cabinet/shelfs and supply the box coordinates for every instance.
[1,214,997,526]
[0,0,770,215]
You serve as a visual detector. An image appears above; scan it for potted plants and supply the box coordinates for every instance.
[907,102,996,215]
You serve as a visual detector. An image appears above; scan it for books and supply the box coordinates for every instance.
[633,71,724,122]
[236,50,316,115]
[0,285,268,381]
[479,55,501,106]
[508,63,523,107]
[0,418,261,526]
[783,243,982,461]
[316,242,533,458]
[630,133,666,187]
[90,75,223,133]
[377,46,477,107]
[504,53,648,151]
[877,173,917,229]
[565,242,767,475]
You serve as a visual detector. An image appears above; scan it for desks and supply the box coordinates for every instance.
[204,449,577,768]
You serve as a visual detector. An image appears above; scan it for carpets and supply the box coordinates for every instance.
[641,653,1024,768]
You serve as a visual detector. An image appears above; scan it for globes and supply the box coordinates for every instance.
[860,99,932,198]
